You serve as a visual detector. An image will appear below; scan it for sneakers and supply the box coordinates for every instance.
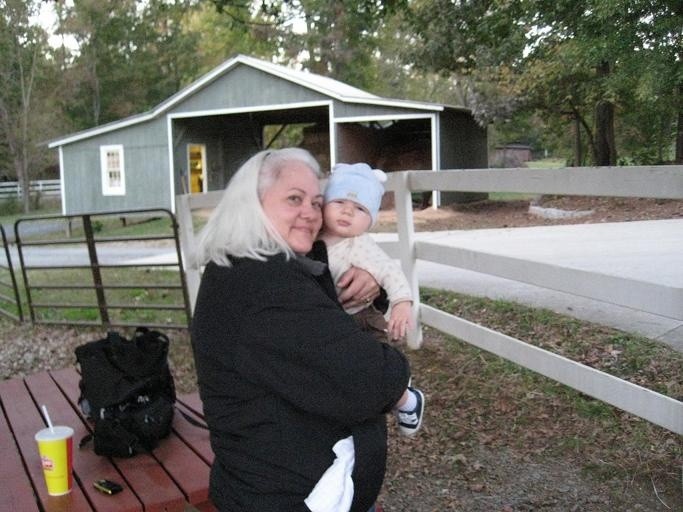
[398,387,425,436]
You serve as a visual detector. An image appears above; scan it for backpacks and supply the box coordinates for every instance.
[73,326,177,459]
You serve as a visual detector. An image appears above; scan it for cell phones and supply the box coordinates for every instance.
[92,478,124,496]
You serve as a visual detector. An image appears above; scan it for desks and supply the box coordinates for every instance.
[0,364,217,512]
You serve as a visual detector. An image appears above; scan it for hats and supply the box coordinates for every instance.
[323,162,388,232]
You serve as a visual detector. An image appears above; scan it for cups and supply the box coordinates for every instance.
[34,425,74,496]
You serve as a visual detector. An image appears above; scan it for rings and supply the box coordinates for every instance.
[363,295,370,304]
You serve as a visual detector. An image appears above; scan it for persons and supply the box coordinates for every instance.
[314,162,426,436]
[186,147,411,511]
